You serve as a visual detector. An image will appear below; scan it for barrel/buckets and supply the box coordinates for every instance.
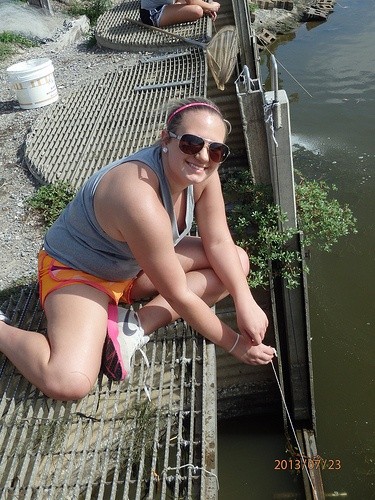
[6,58,60,110]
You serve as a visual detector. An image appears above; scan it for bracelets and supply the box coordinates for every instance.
[229,333,239,353]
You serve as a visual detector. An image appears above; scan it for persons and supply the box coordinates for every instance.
[0,97,278,401]
[139,0,221,27]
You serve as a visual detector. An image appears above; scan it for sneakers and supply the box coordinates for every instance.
[102,303,154,381]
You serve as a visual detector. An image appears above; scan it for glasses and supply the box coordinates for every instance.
[167,130,230,163]
